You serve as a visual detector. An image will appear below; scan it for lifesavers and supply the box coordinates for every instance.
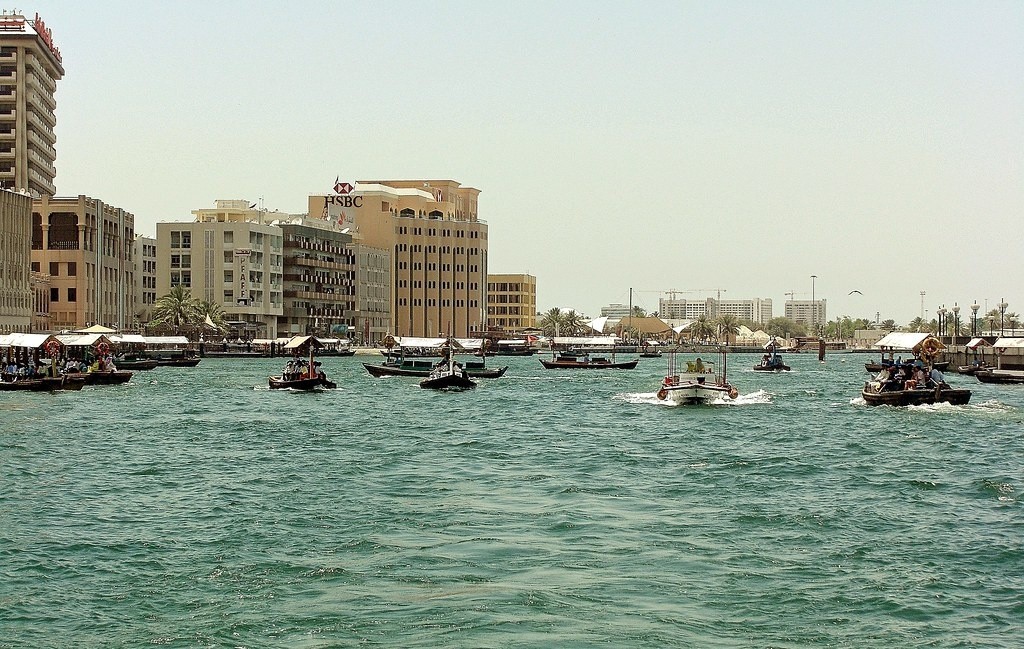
[47,341,60,356]
[999,348,1005,352]
[98,342,109,354]
[762,361,767,367]
[922,338,940,355]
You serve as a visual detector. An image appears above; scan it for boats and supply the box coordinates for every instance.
[538,348,638,370]
[0,334,202,392]
[656,322,738,404]
[974,367,1024,385]
[361,321,510,379]
[865,360,950,375]
[268,336,327,392]
[640,349,663,358]
[861,384,972,406]
[753,336,791,371]
[420,320,477,391]
[958,363,999,377]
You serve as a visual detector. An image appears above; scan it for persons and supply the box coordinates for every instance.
[760,355,770,367]
[395,357,401,368]
[897,356,901,364]
[875,364,944,392]
[642,345,647,354]
[658,349,661,354]
[692,358,704,373]
[972,358,979,365]
[409,347,426,355]
[777,355,785,366]
[429,361,463,378]
[584,354,593,364]
[608,354,615,363]
[283,360,326,381]
[6,355,117,382]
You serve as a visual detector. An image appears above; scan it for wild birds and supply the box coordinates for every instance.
[848,290,863,296]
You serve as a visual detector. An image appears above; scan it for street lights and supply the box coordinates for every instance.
[936,297,1018,337]
[809,275,818,325]
[919,289,928,318]
